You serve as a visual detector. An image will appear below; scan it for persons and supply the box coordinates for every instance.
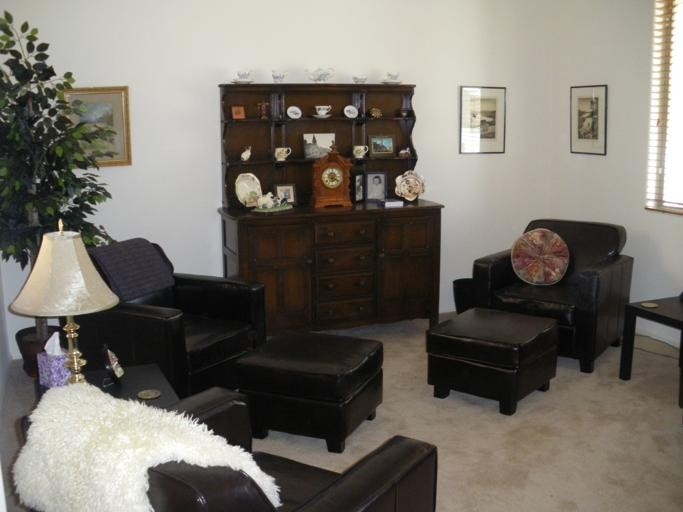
[367,175,382,198]
[580,99,597,139]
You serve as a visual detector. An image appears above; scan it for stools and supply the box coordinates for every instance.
[235,330,385,452]
[427,307,559,414]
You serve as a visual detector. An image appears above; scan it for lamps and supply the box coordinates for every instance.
[7,220,121,382]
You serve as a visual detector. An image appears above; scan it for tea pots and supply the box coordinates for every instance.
[301,62,335,80]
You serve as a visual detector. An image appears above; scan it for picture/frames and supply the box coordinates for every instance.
[570,85,606,155]
[460,87,505,153]
[56,87,131,167]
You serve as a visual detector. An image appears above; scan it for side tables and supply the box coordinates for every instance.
[617,297,680,407]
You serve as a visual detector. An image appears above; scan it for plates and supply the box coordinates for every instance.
[393,170,424,200]
[311,114,331,118]
[235,172,260,206]
[344,105,357,118]
[286,106,301,118]
[229,79,254,85]
[380,80,405,85]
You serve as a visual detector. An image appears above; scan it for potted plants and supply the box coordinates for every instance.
[0,11,113,377]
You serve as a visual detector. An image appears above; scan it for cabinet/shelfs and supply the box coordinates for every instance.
[219,82,444,332]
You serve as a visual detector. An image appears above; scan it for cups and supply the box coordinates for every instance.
[312,105,331,114]
[274,147,291,161]
[352,145,368,158]
[386,71,399,80]
[272,68,285,81]
[234,71,251,79]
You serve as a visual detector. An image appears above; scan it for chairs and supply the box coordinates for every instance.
[79,235,265,398]
[20,386,436,510]
[452,218,633,372]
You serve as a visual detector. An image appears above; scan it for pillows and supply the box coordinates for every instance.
[511,229,569,287]
